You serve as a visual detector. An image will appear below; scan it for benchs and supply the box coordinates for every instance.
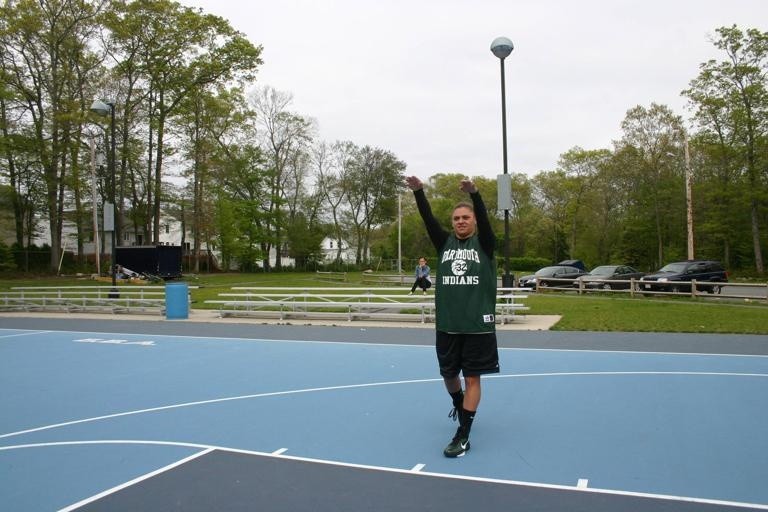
[0,284,200,323]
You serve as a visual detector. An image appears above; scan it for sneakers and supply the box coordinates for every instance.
[422,291,427,296]
[447,401,465,426]
[408,291,414,296]
[442,432,472,457]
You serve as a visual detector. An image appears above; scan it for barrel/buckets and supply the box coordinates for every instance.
[164,282,189,319]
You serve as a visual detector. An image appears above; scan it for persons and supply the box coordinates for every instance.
[408,256,431,295]
[406,176,501,458]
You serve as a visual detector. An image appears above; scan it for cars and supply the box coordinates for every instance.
[573,263,648,295]
[517,264,589,293]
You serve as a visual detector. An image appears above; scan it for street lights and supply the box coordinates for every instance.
[488,35,517,315]
[89,99,121,300]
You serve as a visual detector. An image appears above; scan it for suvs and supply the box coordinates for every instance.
[637,258,731,298]
